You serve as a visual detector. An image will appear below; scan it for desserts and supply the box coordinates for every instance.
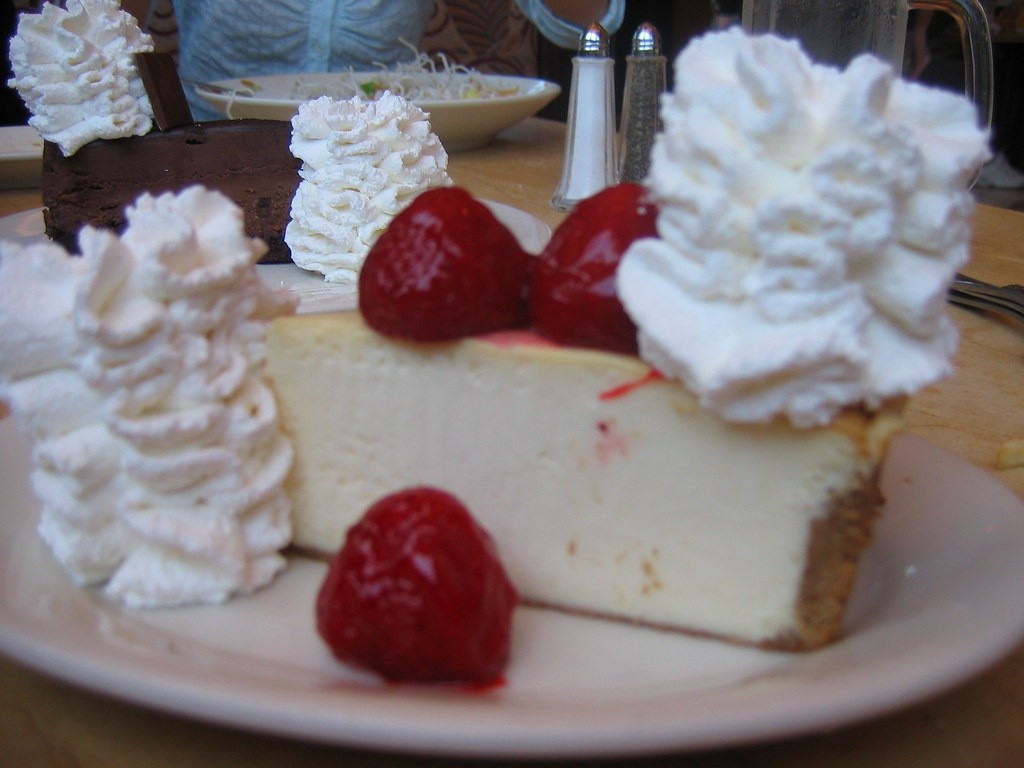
[0,189,295,607]
[286,92,453,283]
[7,0,302,265]
[272,33,989,646]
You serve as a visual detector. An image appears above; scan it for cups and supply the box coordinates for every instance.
[741,0,994,193]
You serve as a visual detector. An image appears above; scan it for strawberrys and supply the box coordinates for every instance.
[315,488,513,687]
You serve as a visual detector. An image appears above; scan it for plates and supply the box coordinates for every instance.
[200,75,560,148]
[0,197,554,398]
[0,366,1024,750]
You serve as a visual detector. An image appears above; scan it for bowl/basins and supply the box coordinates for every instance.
[0,125,43,189]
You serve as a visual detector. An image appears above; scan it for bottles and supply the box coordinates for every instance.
[619,22,667,184]
[550,23,619,213]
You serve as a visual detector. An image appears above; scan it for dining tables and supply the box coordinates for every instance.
[0,116,1024,768]
